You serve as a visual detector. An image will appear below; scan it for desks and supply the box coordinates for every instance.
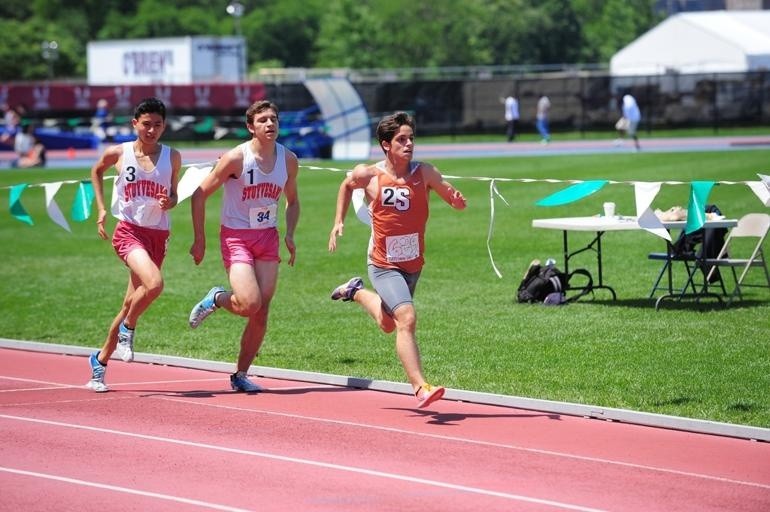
[532,214,736,307]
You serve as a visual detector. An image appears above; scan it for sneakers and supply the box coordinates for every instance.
[229,372,262,393]
[188,287,225,327]
[330,277,364,301]
[118,323,134,361]
[89,352,109,392]
[414,383,444,409]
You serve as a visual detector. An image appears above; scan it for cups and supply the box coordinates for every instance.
[603,202,615,219]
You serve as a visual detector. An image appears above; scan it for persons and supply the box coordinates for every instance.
[504,91,523,142]
[327,109,469,410]
[14,124,36,157]
[0,103,21,145]
[534,92,553,144]
[189,100,302,393]
[615,85,642,153]
[90,97,183,393]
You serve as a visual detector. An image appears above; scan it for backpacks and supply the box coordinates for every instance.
[516,260,594,306]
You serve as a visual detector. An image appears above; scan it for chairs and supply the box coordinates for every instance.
[647,206,729,300]
[696,213,770,308]
